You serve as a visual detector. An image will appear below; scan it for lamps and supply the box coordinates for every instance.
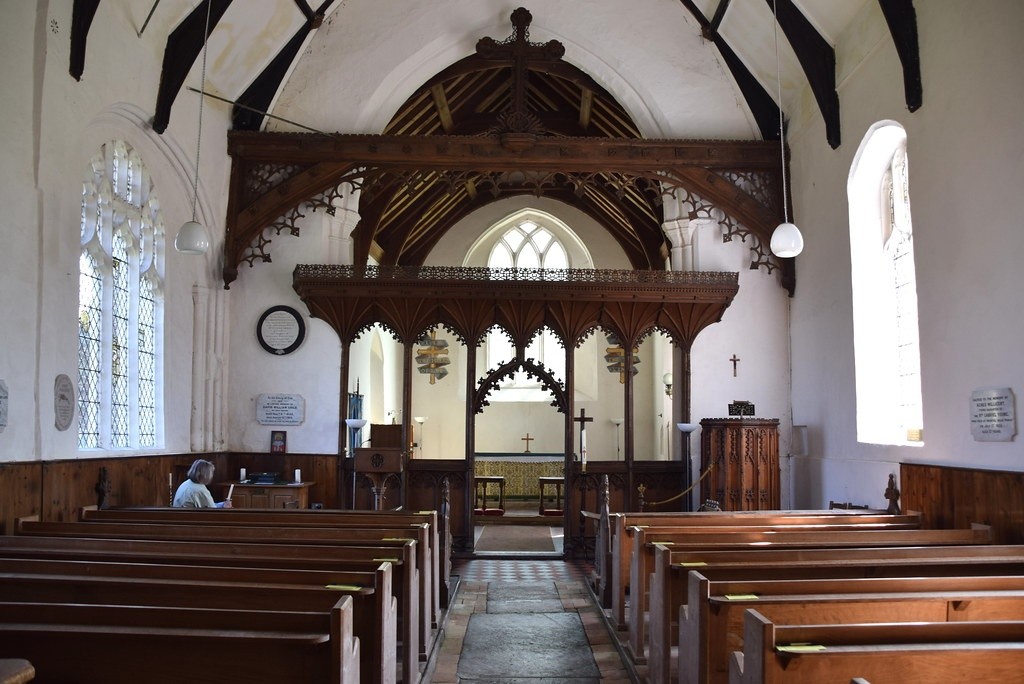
[175,0,212,254]
[414,416,429,459]
[770,0,804,258]
[345,419,367,510]
[663,373,673,401]
[610,418,624,461]
[677,424,698,512]
[787,425,809,458]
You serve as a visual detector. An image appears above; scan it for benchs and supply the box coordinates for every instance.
[612,509,1024,684]
[0,505,452,684]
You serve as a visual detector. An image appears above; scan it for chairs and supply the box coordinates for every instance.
[829,501,847,510]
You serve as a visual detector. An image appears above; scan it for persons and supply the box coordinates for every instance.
[173,459,232,509]
[272,433,284,451]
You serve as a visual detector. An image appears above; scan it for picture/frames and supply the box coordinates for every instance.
[271,430,287,453]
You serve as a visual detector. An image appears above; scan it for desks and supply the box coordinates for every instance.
[217,481,317,509]
[474,475,505,517]
[539,476,564,516]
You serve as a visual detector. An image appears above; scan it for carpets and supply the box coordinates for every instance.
[474,524,556,552]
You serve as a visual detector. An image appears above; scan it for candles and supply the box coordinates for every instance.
[240,467,246,481]
[581,428,587,472]
[227,484,234,499]
[169,472,173,489]
[295,469,301,483]
